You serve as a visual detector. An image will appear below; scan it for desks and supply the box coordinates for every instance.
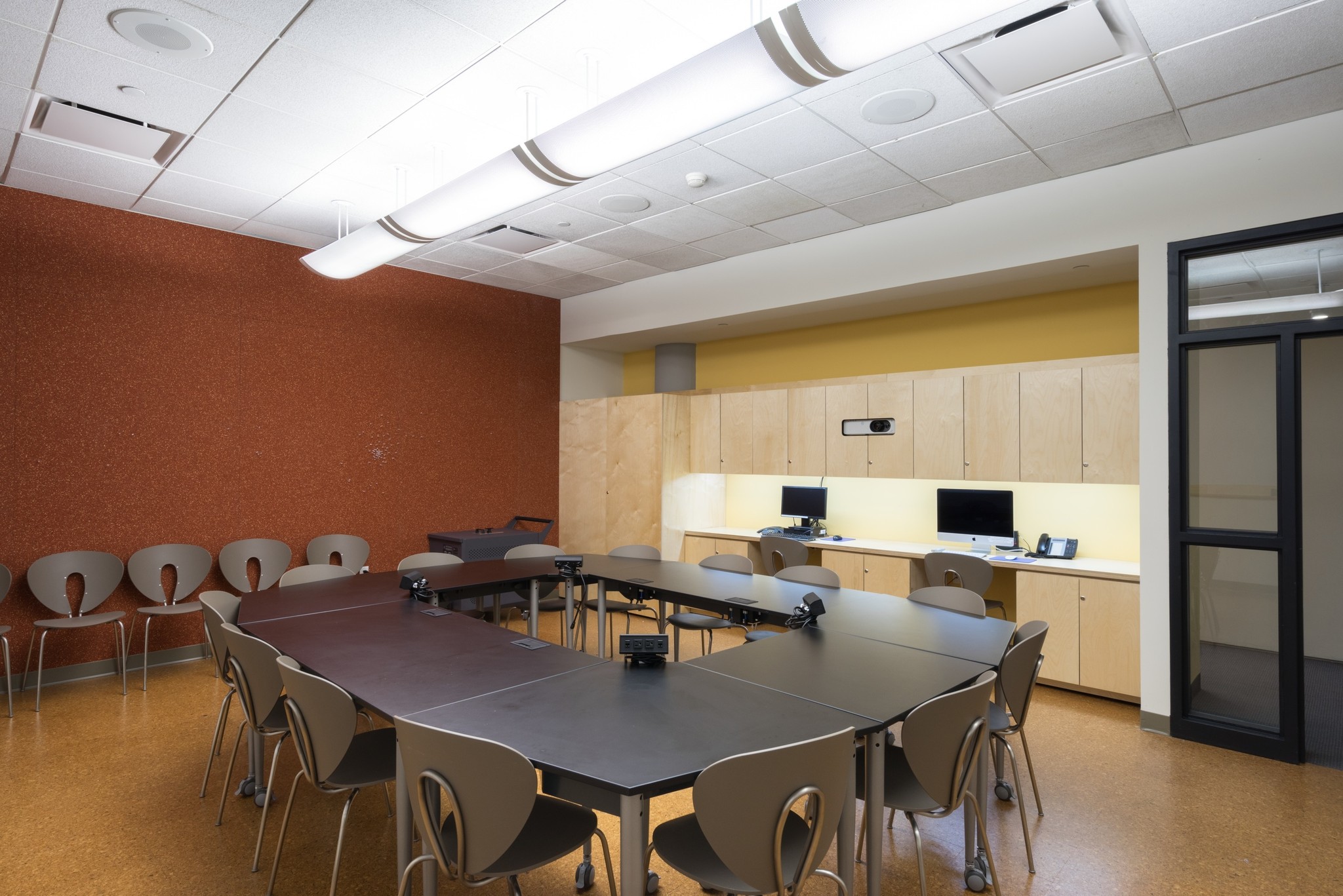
[240,552,1019,894]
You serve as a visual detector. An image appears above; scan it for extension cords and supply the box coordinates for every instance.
[619,634,669,654]
[399,570,424,590]
[802,592,827,617]
[554,555,583,567]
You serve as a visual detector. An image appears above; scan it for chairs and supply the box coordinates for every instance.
[398,552,486,621]
[219,538,292,601]
[280,563,355,588]
[306,533,371,576]
[887,620,1049,873]
[502,543,586,654]
[0,563,15,718]
[120,544,211,692]
[572,544,663,658]
[760,535,809,576]
[221,621,393,873]
[19,549,127,711]
[199,592,246,795]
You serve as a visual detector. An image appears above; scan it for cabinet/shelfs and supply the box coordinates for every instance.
[683,527,1141,709]
[687,355,1138,485]
[558,393,726,623]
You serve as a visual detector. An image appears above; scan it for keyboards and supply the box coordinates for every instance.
[760,532,817,541]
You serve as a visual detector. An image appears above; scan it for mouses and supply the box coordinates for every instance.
[833,535,842,540]
[1005,555,1017,560]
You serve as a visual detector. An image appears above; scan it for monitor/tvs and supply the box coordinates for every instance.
[781,485,827,519]
[936,488,1014,547]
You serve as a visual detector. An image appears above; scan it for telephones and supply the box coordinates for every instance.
[1036,533,1078,559]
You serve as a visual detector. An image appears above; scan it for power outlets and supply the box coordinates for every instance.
[359,566,369,574]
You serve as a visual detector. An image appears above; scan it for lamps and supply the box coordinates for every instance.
[300,0,1052,284]
